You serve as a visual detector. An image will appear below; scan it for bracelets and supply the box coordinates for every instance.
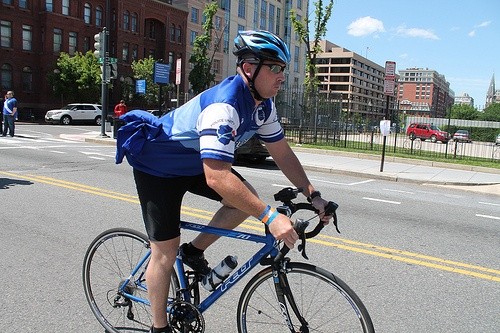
[258,204,279,225]
[307,191,321,202]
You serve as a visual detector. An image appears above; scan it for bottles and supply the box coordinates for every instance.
[201,256,238,291]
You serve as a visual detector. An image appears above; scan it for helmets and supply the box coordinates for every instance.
[233,29,291,65]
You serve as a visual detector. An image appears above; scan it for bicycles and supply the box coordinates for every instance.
[81,186,375,333]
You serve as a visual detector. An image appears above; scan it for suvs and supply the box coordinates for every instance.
[406,123,451,144]
[44,102,102,126]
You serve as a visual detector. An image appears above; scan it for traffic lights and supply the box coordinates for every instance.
[92,32,103,59]
[109,63,116,78]
[99,65,103,79]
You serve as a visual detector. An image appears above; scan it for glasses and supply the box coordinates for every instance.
[243,62,287,74]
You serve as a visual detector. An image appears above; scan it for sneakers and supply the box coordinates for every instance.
[178,243,211,275]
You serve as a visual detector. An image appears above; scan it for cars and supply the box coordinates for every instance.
[452,130,472,143]
[146,109,165,118]
[495,133,500,145]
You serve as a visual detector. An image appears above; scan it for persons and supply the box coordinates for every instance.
[115,29,334,333]
[0,92,5,135]
[114,99,127,139]
[5,94,11,135]
[0,90,17,137]
